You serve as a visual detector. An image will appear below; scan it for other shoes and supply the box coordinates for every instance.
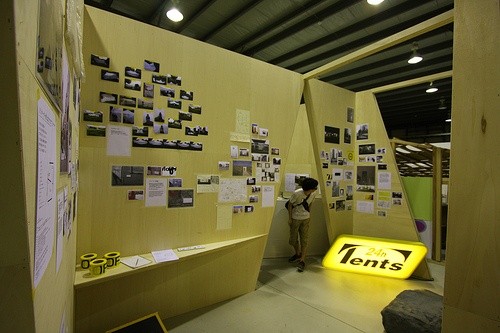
[288,253,301,263]
[297,262,305,270]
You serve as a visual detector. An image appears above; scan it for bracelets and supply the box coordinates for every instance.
[289,218,292,219]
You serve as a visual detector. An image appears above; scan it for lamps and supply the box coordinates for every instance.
[408,45,424,64]
[425,81,438,93]
[165,0,184,22]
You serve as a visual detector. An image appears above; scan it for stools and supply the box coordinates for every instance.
[381,290,443,333]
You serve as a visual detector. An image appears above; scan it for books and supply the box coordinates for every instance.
[152,249,178,263]
[122,255,152,269]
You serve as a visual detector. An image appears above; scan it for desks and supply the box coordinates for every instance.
[74,234,268,290]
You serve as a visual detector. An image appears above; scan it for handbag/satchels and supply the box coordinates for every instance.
[284,201,293,210]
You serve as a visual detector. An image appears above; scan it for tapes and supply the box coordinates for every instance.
[90,259,107,276]
[104,252,120,268]
[80,253,97,270]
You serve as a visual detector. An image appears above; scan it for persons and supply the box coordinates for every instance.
[285,178,318,271]
